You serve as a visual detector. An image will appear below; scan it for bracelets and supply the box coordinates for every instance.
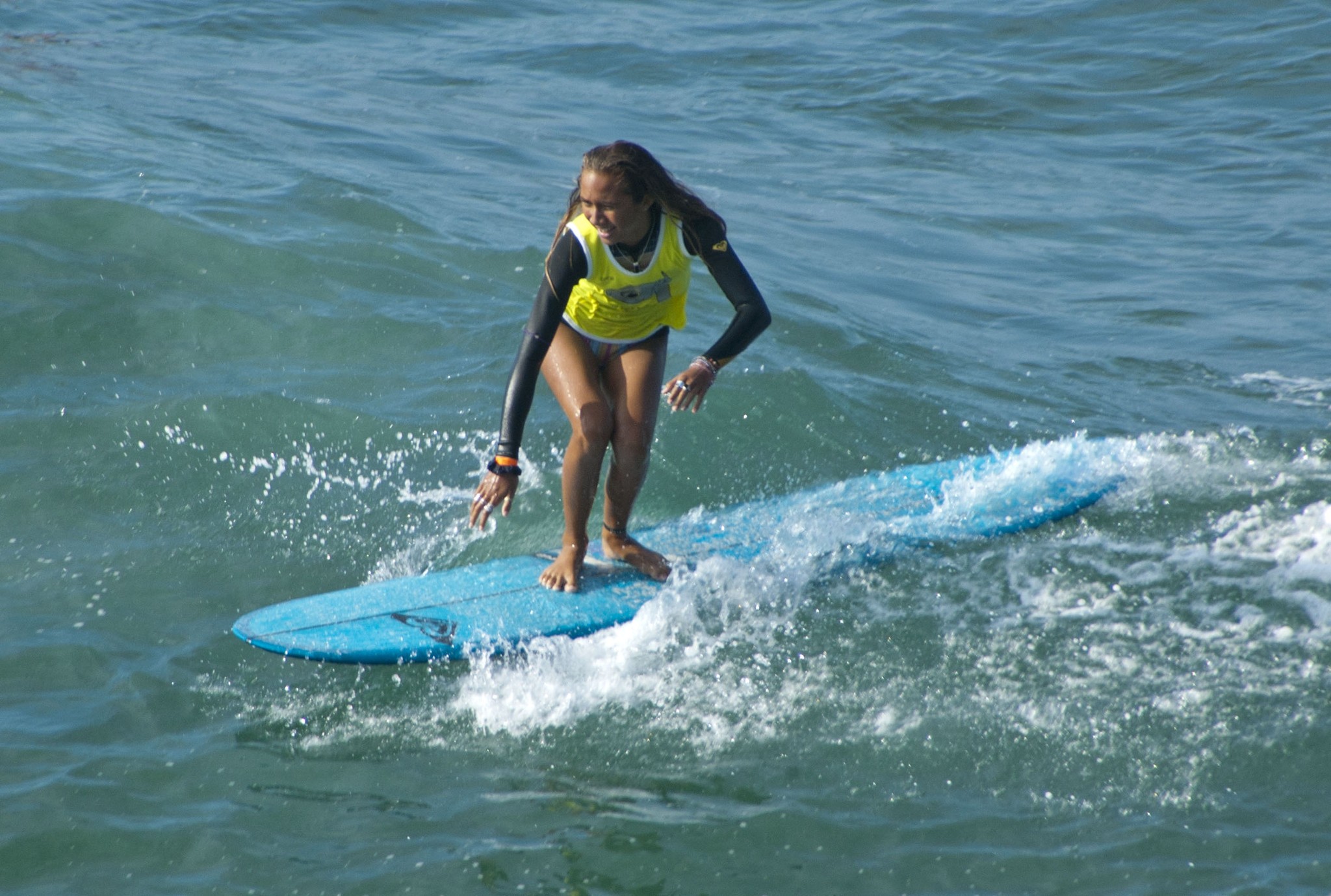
[495,450,519,459]
[695,359,716,374]
[690,362,713,376]
[693,355,717,372]
[487,458,522,476]
[498,440,521,447]
[495,456,517,466]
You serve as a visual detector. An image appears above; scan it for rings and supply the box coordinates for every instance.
[683,384,691,391]
[675,381,685,389]
[484,503,494,513]
[474,494,481,501]
[504,497,512,501]
[480,498,486,505]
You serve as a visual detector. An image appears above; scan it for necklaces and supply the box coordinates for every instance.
[614,212,655,273]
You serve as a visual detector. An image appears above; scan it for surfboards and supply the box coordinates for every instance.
[230,435,1143,664]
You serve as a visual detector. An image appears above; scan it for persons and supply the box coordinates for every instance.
[469,142,772,592]
